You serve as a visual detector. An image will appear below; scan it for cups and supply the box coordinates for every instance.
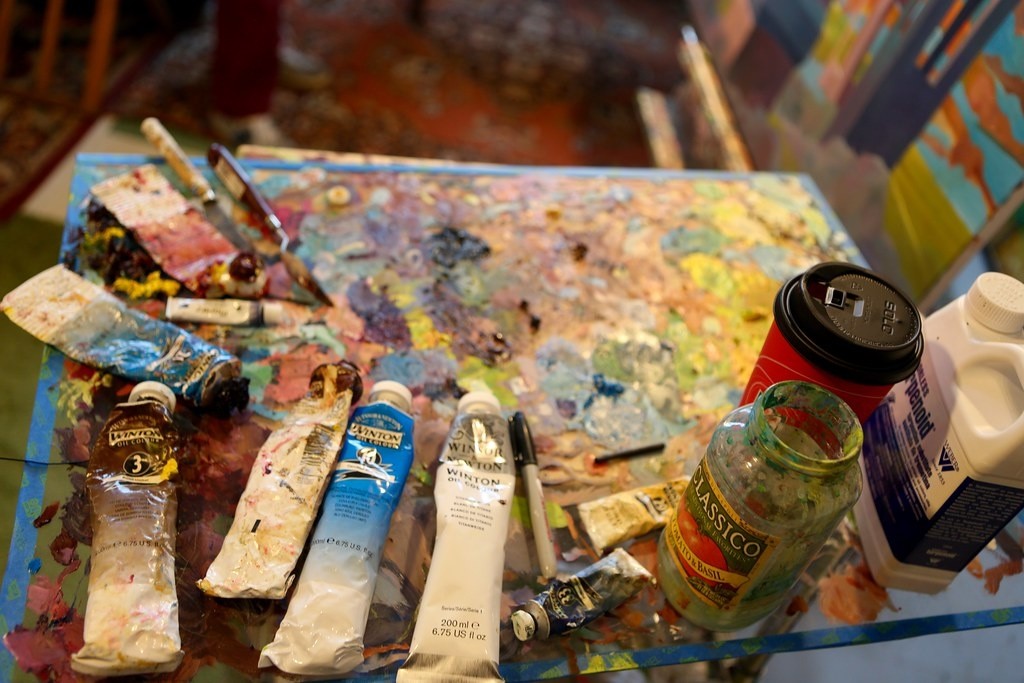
[739,263,924,427]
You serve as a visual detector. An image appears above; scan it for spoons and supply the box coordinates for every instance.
[208,144,335,308]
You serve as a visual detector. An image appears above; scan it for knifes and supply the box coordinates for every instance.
[141,118,254,255]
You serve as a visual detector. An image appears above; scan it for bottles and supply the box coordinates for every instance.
[854,271,1024,594]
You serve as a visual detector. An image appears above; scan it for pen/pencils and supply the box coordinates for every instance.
[507,411,559,582]
